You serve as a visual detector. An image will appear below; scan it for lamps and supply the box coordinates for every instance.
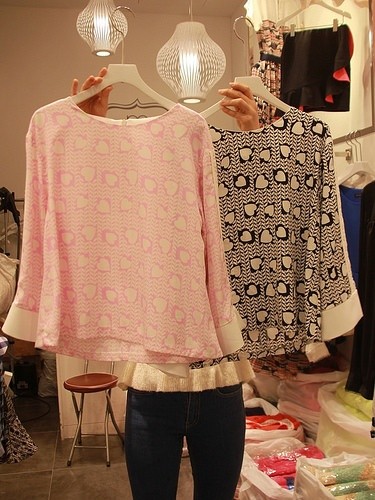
[76,0,129,58]
[156,0,226,105]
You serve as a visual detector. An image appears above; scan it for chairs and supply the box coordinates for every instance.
[64,360,126,467]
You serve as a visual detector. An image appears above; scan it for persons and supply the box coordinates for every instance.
[71,67,260,500]
[0,252,38,465]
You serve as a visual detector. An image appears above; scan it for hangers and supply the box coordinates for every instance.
[199,15,291,119]
[276,0,352,37]
[337,130,375,188]
[72,5,177,112]
